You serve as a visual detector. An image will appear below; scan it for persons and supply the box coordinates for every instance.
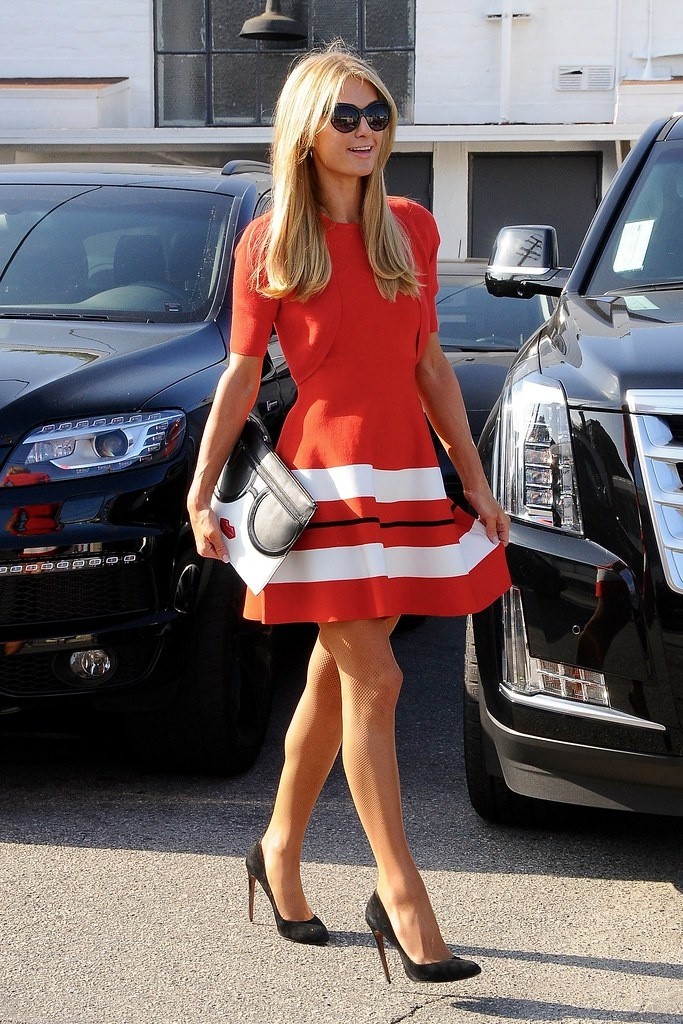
[186,39,514,983]
[572,403,648,670]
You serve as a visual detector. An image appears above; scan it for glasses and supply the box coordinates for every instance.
[322,100,391,133]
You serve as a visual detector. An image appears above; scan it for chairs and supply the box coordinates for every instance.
[439,322,467,339]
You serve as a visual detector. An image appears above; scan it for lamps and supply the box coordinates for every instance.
[238,0,308,42]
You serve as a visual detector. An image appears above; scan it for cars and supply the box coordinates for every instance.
[462,110,683,826]
[419,272,562,513]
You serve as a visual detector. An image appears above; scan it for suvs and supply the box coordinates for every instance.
[1,158,383,779]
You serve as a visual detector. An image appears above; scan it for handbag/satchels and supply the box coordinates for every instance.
[209,418,319,597]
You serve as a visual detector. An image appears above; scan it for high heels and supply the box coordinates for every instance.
[245,840,330,945]
[365,887,481,986]
